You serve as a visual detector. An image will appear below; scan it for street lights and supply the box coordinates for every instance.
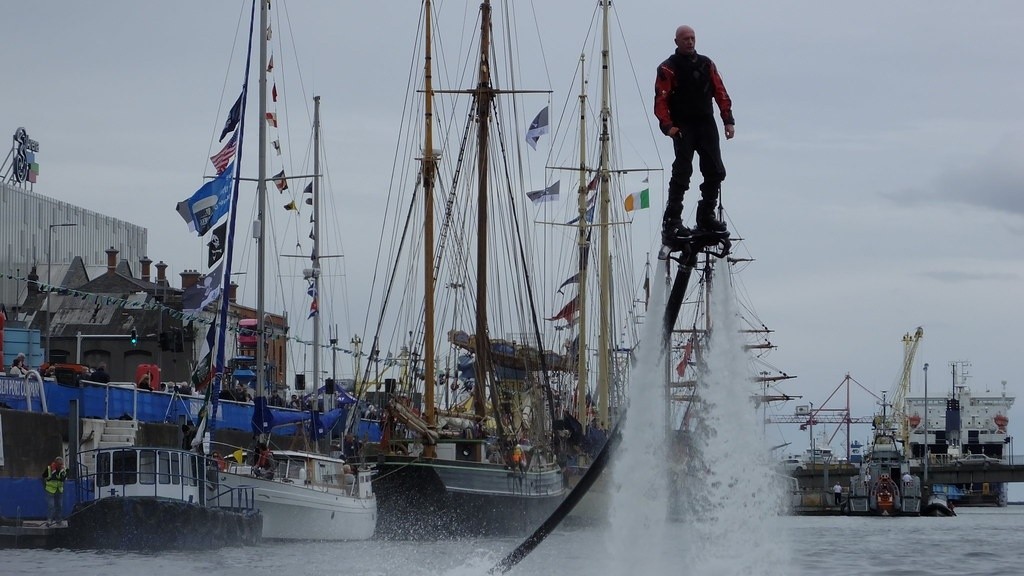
[921,363,929,483]
[44,223,77,363]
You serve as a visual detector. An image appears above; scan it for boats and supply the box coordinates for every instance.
[921,491,958,517]
[904,362,1017,508]
[840,389,921,516]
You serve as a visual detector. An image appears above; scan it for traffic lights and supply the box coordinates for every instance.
[131,330,137,343]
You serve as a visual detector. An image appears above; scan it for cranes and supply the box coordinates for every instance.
[766,375,909,463]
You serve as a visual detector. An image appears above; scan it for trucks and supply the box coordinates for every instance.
[3,328,41,376]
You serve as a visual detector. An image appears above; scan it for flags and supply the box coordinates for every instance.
[526,105,692,378]
[174,3,319,389]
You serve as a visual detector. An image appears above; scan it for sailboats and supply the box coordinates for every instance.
[340,0,804,536]
[64,0,380,545]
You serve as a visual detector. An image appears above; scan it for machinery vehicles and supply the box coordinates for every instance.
[850,326,935,467]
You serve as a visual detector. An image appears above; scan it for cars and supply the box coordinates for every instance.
[42,363,96,378]
[950,454,1000,468]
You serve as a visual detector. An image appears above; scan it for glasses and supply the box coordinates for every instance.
[213,455,219,458]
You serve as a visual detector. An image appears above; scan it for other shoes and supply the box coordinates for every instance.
[663,224,693,238]
[696,219,727,234]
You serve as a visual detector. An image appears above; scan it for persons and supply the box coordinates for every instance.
[180,424,205,488]
[41,457,69,528]
[252,442,276,481]
[833,482,842,505]
[654,24,736,238]
[9,353,312,411]
[902,472,913,487]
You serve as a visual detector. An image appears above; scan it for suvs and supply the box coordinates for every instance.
[773,459,808,473]
